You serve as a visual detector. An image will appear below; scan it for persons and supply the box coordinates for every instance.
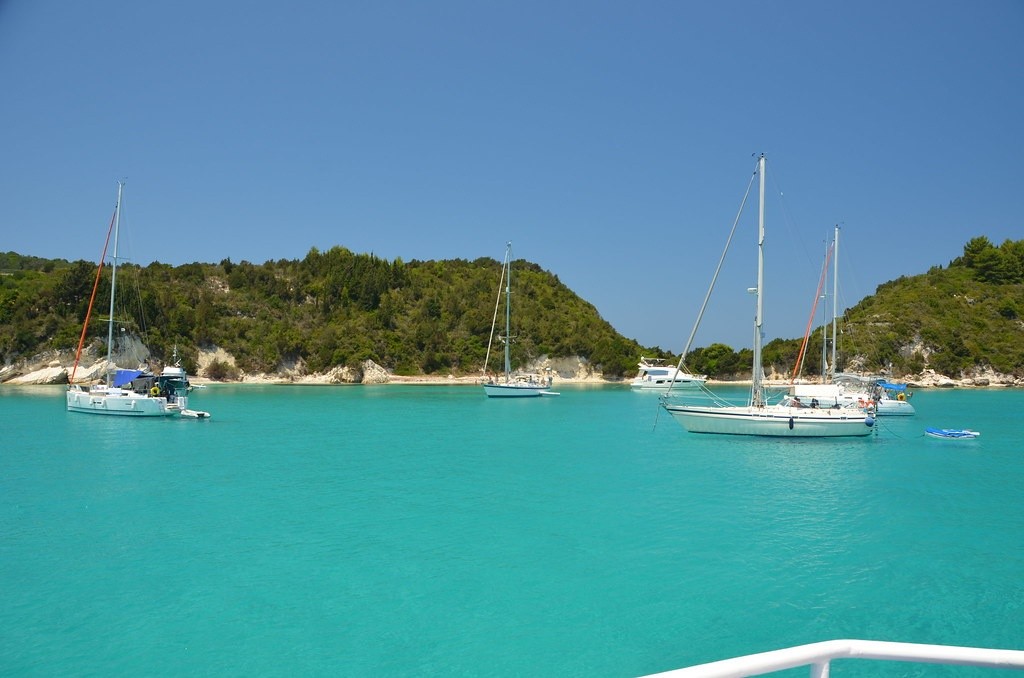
[160,386,177,403]
[790,398,800,407]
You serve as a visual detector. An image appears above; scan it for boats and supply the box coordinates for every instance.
[922,428,981,440]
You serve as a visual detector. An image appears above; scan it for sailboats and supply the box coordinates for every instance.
[654,152,877,437]
[66,179,213,420]
[788,221,918,418]
[477,240,560,397]
[629,366,707,388]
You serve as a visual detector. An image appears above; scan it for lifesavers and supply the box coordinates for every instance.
[151,387,160,395]
[898,394,905,401]
[866,401,875,411]
